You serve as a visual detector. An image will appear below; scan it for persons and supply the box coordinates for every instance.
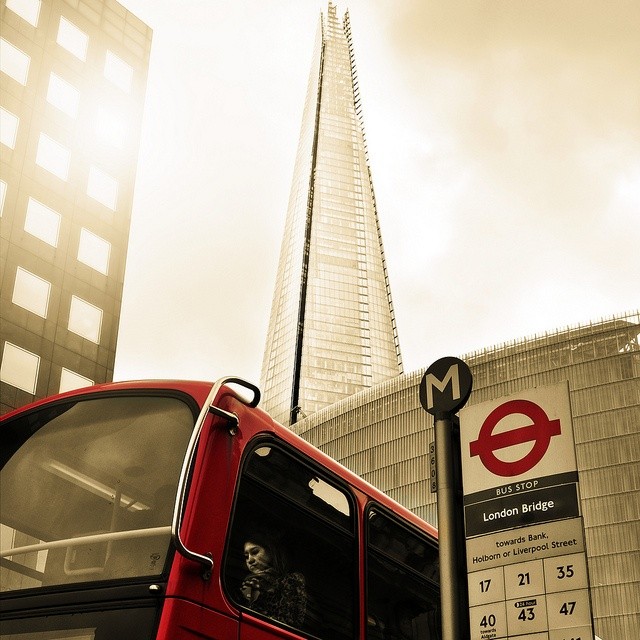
[241,532,314,632]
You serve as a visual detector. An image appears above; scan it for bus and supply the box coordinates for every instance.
[1,378,441,640]
